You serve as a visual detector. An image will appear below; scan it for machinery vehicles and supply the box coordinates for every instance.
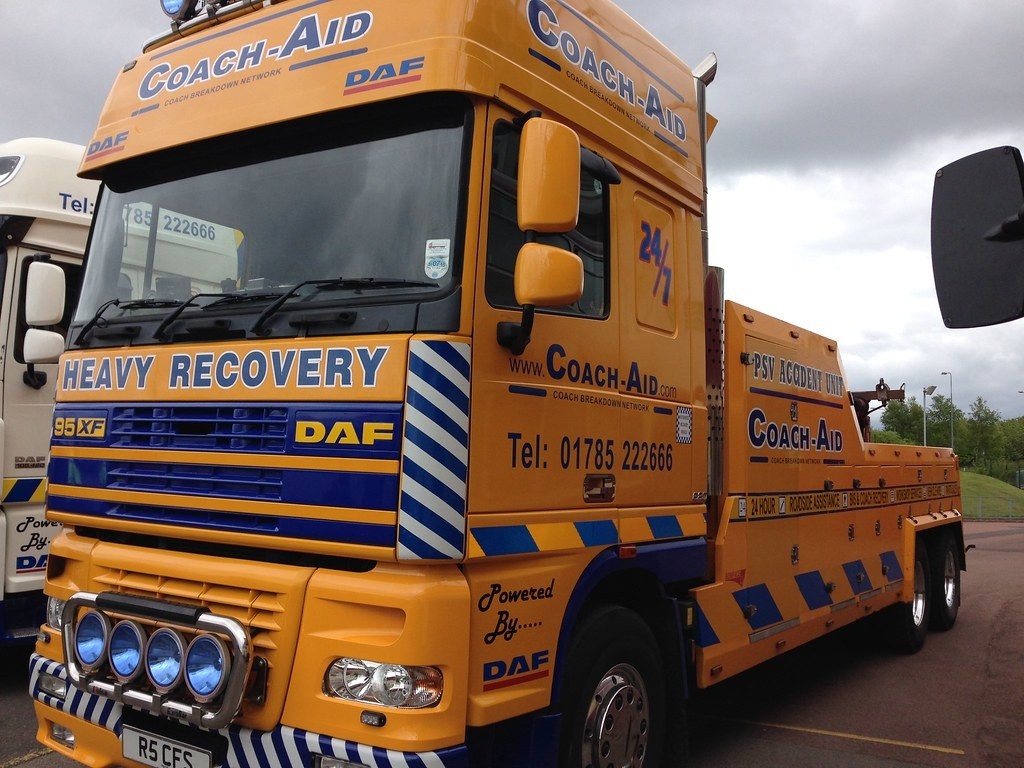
[0,0,975,768]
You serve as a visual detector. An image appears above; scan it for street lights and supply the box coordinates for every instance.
[942,372,954,448]
[922,386,935,446]
